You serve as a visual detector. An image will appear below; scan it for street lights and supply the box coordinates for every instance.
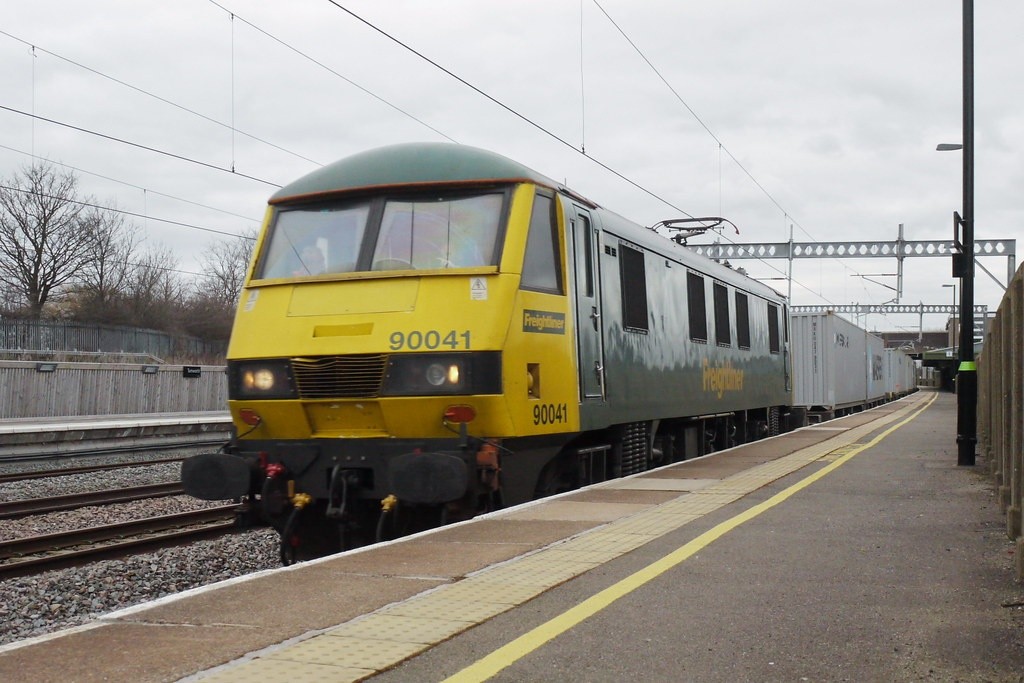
[941,284,957,395]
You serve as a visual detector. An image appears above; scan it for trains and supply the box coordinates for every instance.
[180,141,918,567]
